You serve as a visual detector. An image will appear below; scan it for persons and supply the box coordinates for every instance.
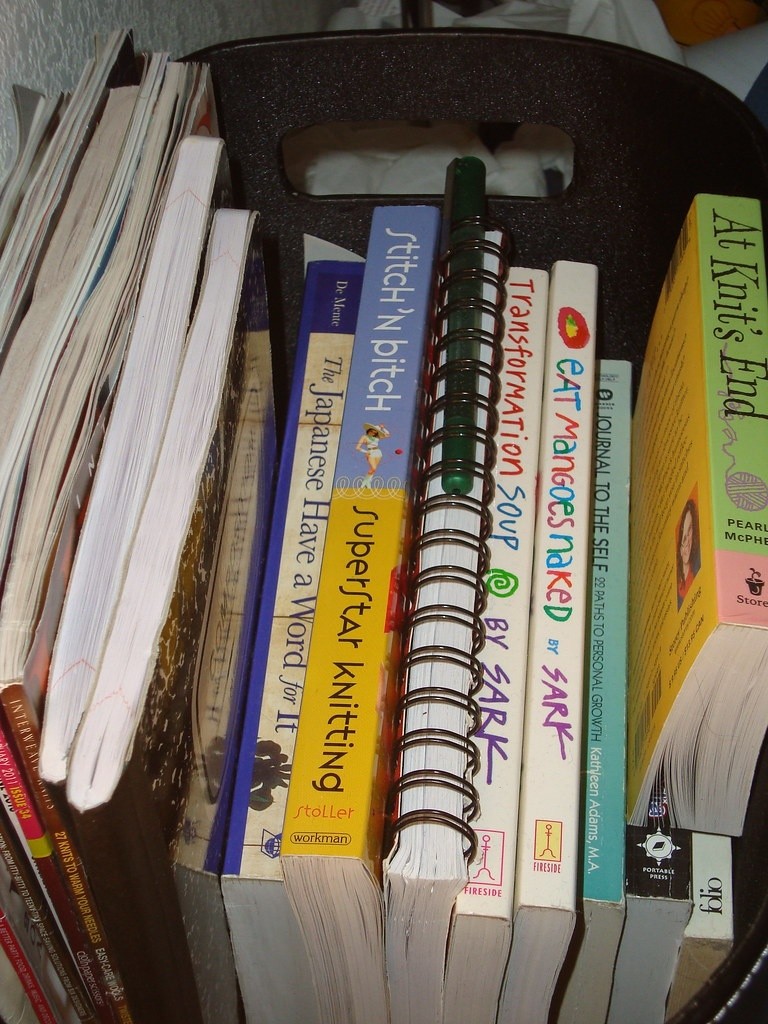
[676,500,701,608]
[357,424,390,489]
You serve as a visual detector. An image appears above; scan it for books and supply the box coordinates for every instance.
[0,30,768,1024]
